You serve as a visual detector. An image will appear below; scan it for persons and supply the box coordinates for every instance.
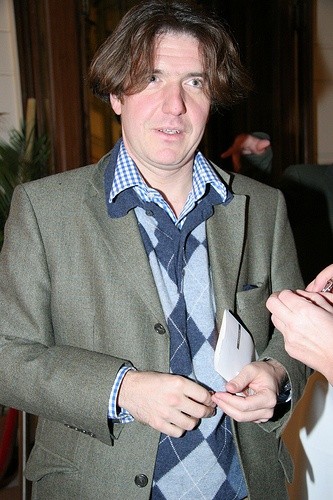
[0,1,306,500]
[206,96,289,183]
[267,259,332,500]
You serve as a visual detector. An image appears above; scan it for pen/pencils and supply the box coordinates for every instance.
[320,278,333,294]
